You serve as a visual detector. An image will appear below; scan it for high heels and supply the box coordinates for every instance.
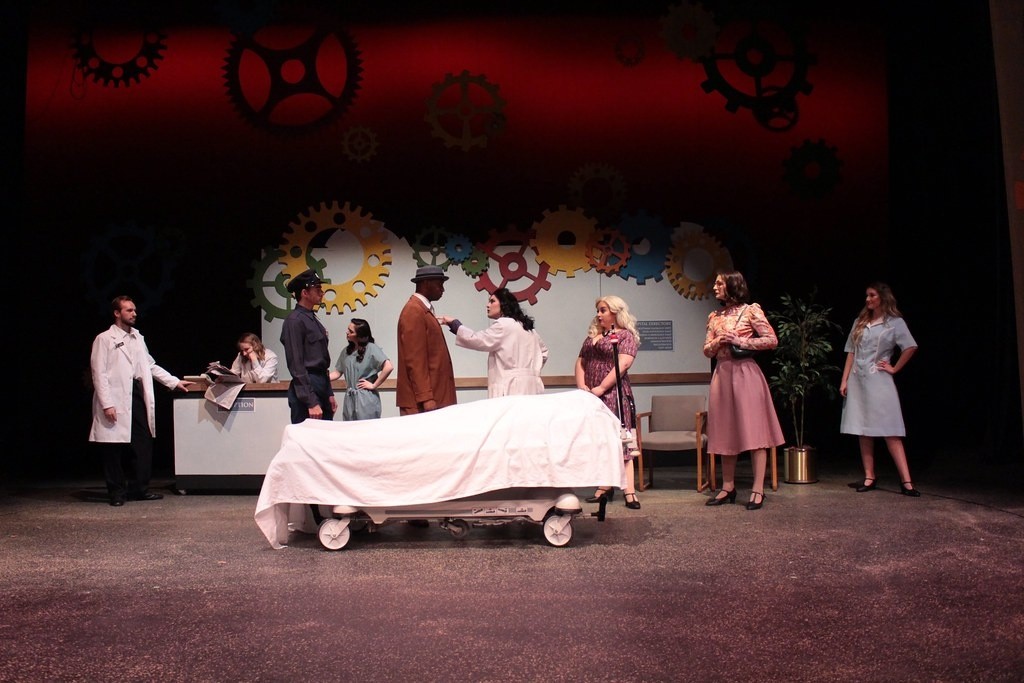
[585,487,614,503]
[705,488,737,506]
[623,492,640,509]
[900,481,920,497]
[857,478,876,492]
[746,490,766,510]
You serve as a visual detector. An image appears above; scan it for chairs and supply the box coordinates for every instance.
[636,395,709,492]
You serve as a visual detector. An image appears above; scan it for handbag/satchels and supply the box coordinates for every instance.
[731,305,755,359]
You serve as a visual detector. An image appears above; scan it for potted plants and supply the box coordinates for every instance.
[767,285,845,484]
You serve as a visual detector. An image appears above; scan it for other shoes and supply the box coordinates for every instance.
[408,520,430,528]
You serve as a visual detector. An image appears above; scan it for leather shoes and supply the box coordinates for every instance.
[136,493,163,500]
[111,496,124,506]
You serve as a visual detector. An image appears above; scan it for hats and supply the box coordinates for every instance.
[287,269,328,293]
[410,265,449,283]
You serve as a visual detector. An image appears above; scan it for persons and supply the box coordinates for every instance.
[704,269,786,509]
[231,333,281,383]
[329,319,394,420]
[575,296,640,510]
[87,296,196,505]
[436,288,549,398]
[281,269,337,423]
[840,283,921,496]
[396,265,456,526]
